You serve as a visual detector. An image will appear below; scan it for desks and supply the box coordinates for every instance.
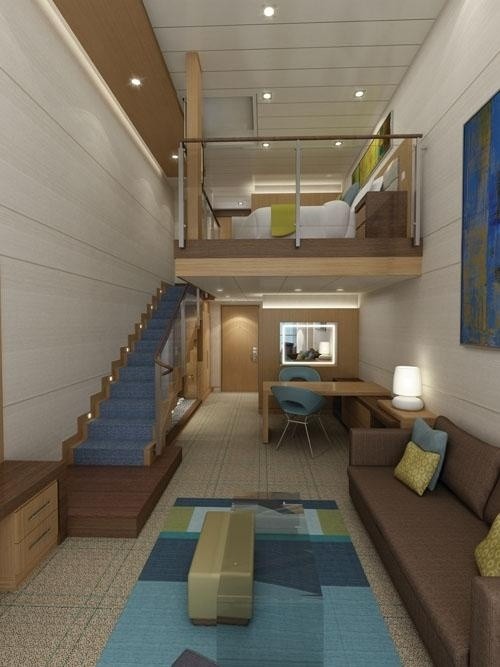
[262,378,438,444]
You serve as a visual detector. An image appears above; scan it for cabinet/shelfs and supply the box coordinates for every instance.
[0,460,66,593]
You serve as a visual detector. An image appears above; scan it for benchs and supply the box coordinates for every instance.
[188,509,255,627]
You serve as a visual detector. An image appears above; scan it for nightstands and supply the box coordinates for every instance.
[354,191,407,239]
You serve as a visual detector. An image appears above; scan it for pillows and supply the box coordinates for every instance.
[473,513,500,577]
[296,351,314,360]
[323,200,349,208]
[395,441,440,496]
[433,415,500,520]
[413,416,448,492]
[483,473,500,526]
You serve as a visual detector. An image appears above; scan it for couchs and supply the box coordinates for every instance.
[315,355,332,361]
[347,427,500,667]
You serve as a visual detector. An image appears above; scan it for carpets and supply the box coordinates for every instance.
[97,498,405,667]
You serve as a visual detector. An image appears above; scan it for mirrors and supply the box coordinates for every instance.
[280,321,338,367]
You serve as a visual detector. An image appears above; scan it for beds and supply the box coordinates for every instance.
[236,183,360,239]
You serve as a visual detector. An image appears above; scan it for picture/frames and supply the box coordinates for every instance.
[460,89,500,349]
[351,111,393,190]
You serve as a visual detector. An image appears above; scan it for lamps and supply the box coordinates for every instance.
[319,341,329,355]
[391,365,424,411]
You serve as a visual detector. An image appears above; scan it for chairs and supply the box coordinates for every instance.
[279,366,322,381]
[271,385,332,458]
